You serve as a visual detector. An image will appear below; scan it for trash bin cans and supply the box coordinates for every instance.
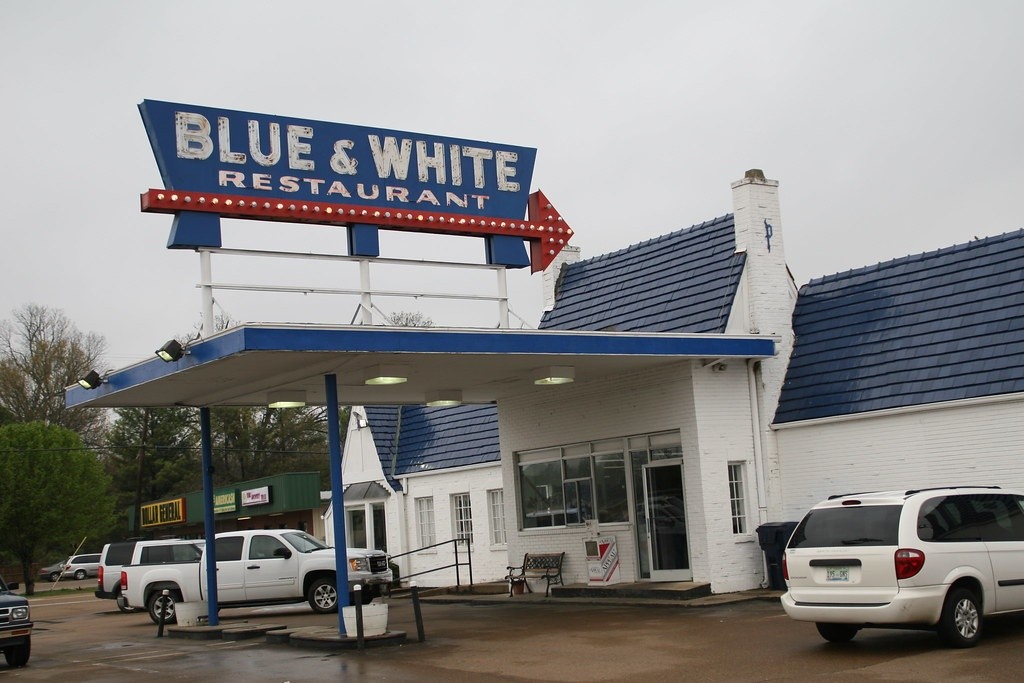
[757,520,799,589]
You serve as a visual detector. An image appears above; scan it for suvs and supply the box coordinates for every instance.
[0,576,34,669]
[94,537,206,613]
[60,553,103,581]
[526,506,588,527]
[637,495,685,539]
[780,484,1024,650]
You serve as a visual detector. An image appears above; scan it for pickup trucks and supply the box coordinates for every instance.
[117,528,393,625]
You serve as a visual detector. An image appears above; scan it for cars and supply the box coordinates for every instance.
[37,560,68,582]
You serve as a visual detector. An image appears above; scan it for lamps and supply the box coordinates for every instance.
[267,391,306,408]
[77,370,105,390]
[154,340,188,363]
[426,391,462,406]
[716,363,728,373]
[532,366,576,385]
[365,365,410,385]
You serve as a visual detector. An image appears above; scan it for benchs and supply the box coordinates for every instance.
[505,550,566,598]
